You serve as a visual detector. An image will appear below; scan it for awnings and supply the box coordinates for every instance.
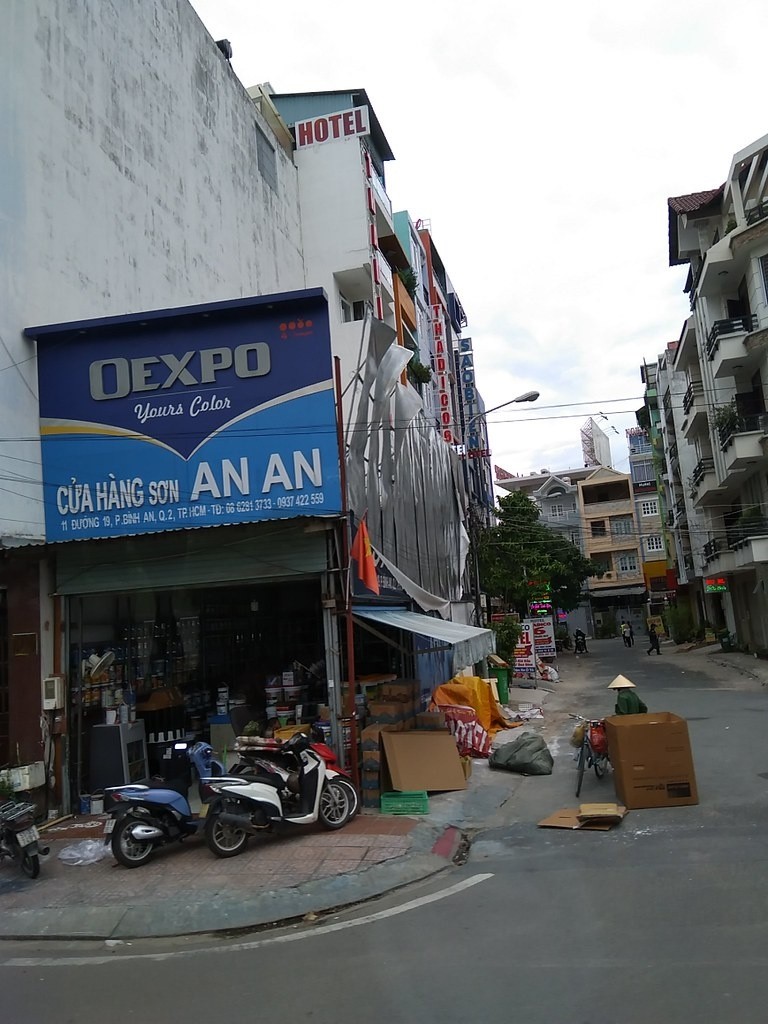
[351,604,495,673]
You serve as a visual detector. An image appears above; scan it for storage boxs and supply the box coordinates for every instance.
[605,713,699,809]
[0,761,45,792]
[273,678,472,814]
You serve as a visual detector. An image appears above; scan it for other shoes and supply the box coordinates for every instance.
[625,642,634,648]
[657,651,662,655]
[647,651,650,655]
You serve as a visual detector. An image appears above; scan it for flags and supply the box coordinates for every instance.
[350,519,379,596]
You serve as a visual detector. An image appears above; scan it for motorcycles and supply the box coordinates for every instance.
[574,634,587,653]
[0,794,50,879]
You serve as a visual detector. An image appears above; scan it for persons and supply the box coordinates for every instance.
[607,674,648,717]
[620,620,634,649]
[573,627,589,654]
[647,623,662,656]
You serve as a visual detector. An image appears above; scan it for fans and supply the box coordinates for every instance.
[80,651,115,679]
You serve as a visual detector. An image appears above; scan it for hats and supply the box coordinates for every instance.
[606,673,636,690]
[624,624,629,628]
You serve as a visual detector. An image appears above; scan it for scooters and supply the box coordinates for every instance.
[102,742,225,868]
[199,718,359,858]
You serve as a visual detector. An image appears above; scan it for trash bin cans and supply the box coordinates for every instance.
[487,667,514,705]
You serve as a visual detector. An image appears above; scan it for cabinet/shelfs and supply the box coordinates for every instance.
[89,720,150,794]
[127,589,398,691]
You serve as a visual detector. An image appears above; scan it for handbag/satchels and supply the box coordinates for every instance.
[570,723,584,748]
[590,721,608,752]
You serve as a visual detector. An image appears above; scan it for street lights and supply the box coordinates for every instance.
[464,392,540,677]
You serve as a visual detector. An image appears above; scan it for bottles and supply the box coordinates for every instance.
[69,604,246,745]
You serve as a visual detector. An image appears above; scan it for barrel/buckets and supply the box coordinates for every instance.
[91,789,104,815]
[265,674,398,782]
[79,790,91,814]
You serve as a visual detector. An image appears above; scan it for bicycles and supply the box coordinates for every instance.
[568,713,612,797]
